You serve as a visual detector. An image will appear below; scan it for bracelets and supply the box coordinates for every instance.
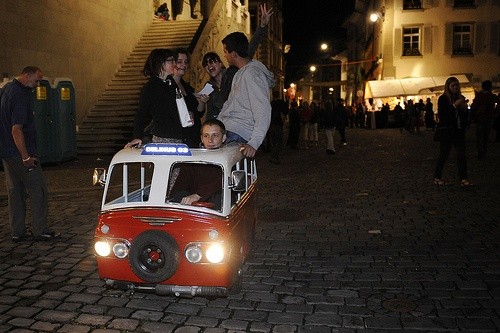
[23,157,30,162]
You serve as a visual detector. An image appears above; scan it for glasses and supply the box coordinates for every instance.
[166,58,174,62]
[202,57,219,68]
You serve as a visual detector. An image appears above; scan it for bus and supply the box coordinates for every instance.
[90,140,260,296]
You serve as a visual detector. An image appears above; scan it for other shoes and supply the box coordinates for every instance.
[460,180,479,189]
[32,231,61,240]
[432,180,452,186]
[12,235,21,242]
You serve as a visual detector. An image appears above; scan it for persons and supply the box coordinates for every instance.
[0,67,61,242]
[123,3,275,205]
[432,77,480,188]
[470,79,500,158]
[381,98,435,134]
[268,91,365,164]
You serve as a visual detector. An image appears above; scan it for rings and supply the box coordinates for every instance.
[29,169,32,172]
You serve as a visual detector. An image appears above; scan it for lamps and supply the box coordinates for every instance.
[370,12,385,22]
